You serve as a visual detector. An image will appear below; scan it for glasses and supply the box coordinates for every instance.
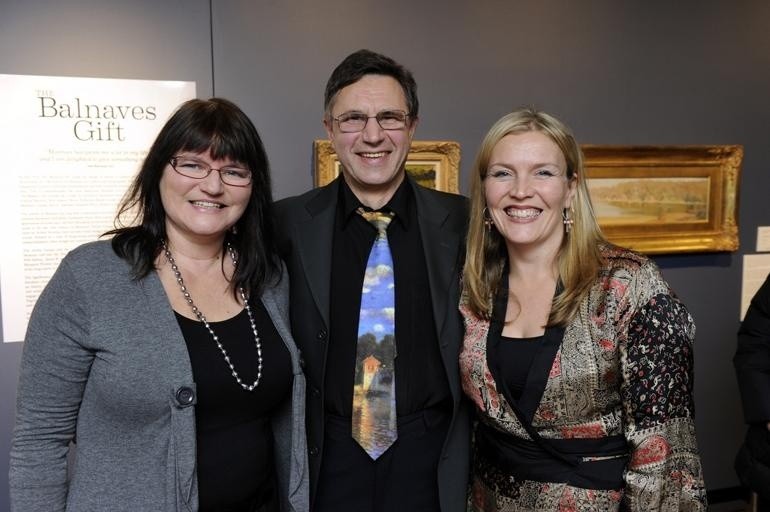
[330,110,411,133]
[168,156,252,187]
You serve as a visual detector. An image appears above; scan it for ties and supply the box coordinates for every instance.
[351,207,398,461]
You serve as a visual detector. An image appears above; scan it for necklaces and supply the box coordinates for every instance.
[160,241,264,392]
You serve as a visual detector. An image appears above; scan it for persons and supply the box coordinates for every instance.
[458,107,708,511]
[266,49,471,512]
[733,273,770,512]
[9,50,309,512]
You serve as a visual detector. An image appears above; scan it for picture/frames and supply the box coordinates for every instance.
[577,144,745,256]
[314,139,462,195]
